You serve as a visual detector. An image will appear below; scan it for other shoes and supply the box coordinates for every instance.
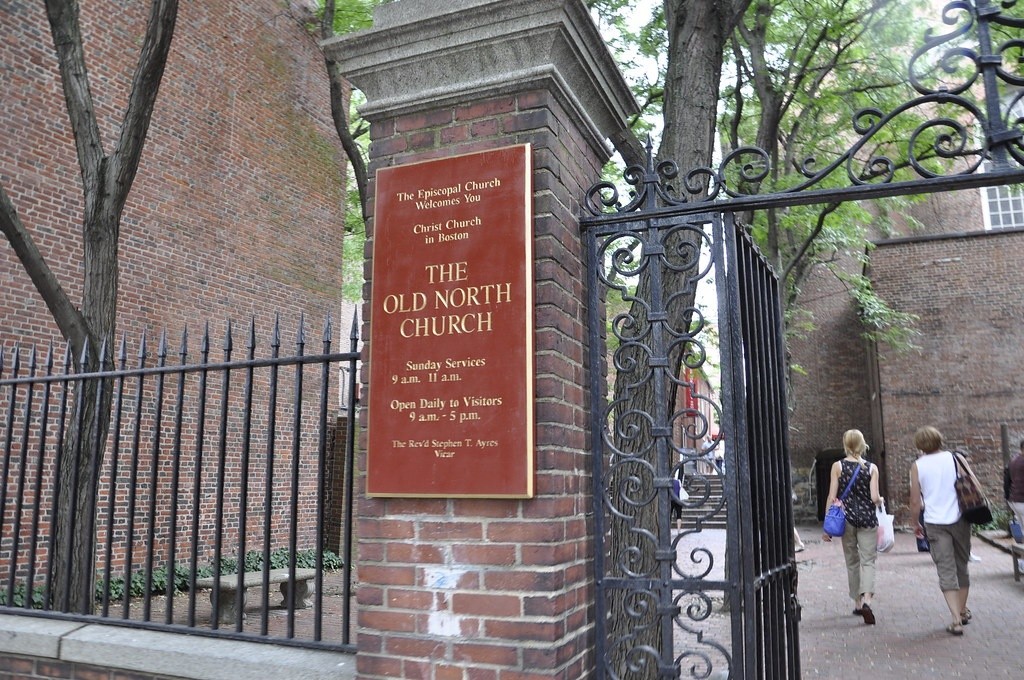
[795,543,805,551]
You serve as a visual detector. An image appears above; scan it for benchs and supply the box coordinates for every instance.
[188,566,325,625]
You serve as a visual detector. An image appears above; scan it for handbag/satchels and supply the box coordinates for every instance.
[678,479,690,502]
[952,452,993,526]
[876,497,895,553]
[823,505,844,537]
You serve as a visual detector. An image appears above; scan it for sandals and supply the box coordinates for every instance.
[853,608,861,615]
[862,603,876,625]
[946,623,964,636]
[960,606,971,624]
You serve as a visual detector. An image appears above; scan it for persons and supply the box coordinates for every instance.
[792,488,805,552]
[702,436,719,474]
[909,452,931,551]
[670,473,682,536]
[910,424,979,635]
[822,429,885,624]
[953,449,981,561]
[1007,439,1024,575]
[715,455,723,475]
